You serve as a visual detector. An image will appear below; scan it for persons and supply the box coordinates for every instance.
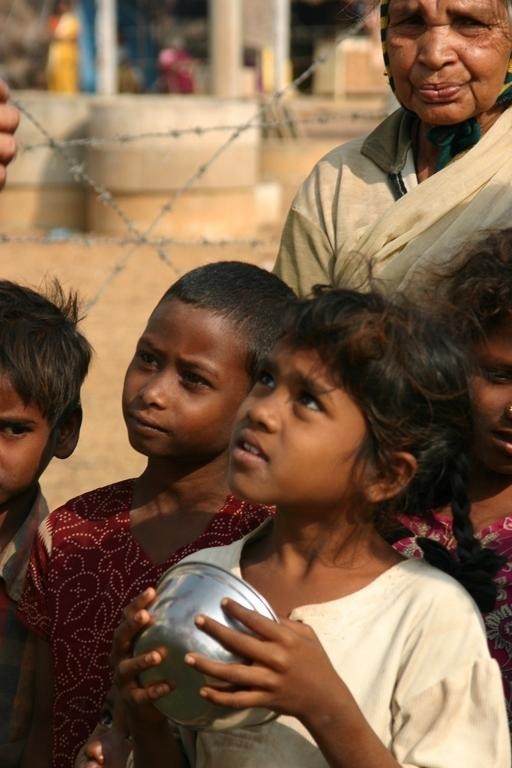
[1,279,92,765]
[111,286,512,766]
[381,223,511,731]
[72,707,187,767]
[0,80,21,188]
[275,1,511,304]
[16,258,299,767]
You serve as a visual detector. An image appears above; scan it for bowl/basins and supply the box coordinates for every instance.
[131,560,285,731]
